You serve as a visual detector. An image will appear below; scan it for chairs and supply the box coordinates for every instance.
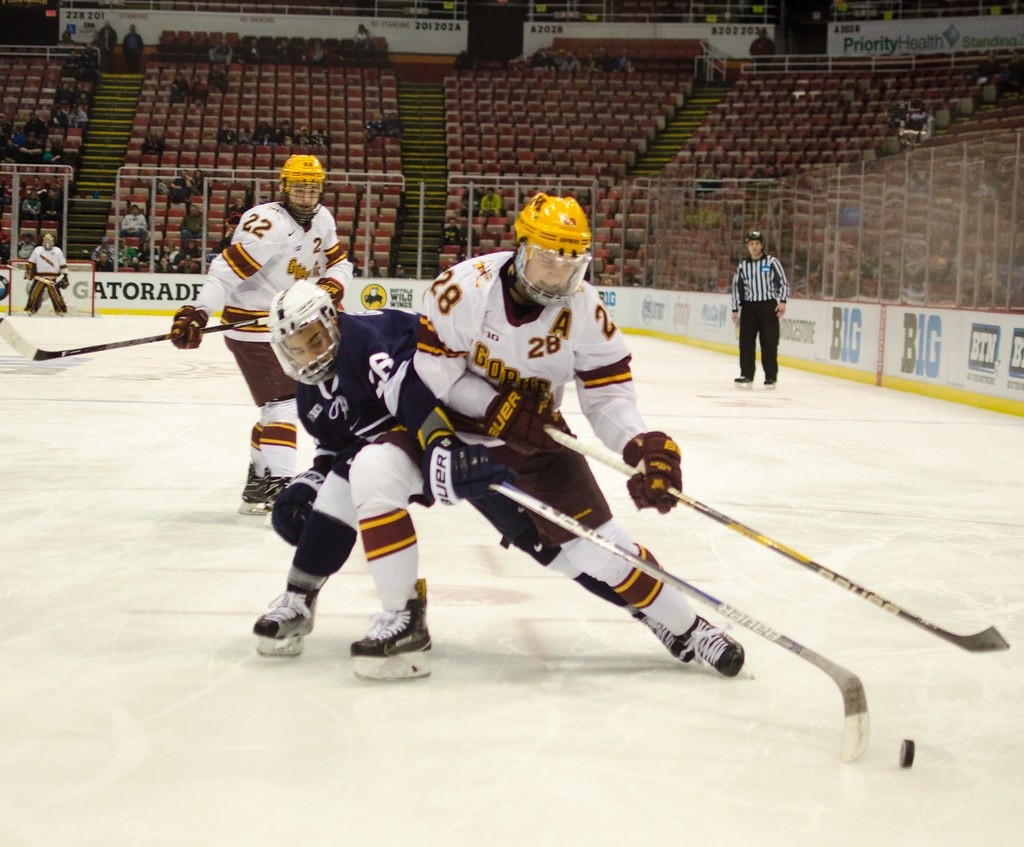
[0,46,95,244]
[105,0,402,278]
[439,0,1024,304]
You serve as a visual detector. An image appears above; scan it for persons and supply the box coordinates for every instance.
[0,0,1024,313]
[350,190,748,678]
[732,229,790,385]
[250,283,698,664]
[168,155,352,513]
[24,232,69,318]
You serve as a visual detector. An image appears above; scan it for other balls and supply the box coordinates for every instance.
[899,739,916,769]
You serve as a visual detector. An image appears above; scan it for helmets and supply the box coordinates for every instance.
[280,154,325,220]
[745,232,763,242]
[266,279,337,385]
[513,192,592,307]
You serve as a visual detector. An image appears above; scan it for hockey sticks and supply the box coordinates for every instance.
[541,422,1012,654]
[6,264,65,287]
[0,315,269,363]
[486,483,871,760]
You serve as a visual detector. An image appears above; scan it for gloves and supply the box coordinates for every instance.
[421,436,518,506]
[272,483,317,547]
[476,391,579,457]
[171,305,208,349]
[316,279,343,305]
[627,432,682,514]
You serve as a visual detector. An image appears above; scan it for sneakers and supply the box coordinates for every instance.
[239,463,281,516]
[252,583,319,657]
[734,376,754,387]
[351,599,433,679]
[764,377,777,388]
[675,615,754,680]
[259,467,295,515]
